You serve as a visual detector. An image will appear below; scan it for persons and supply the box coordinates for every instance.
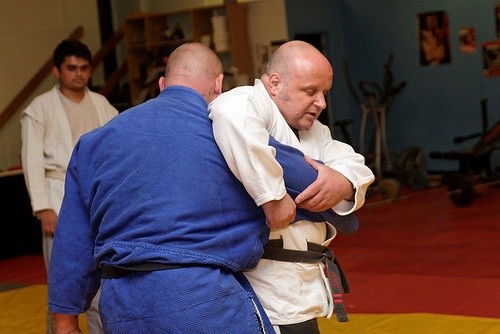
[207,40,375,334]
[19,40,120,334]
[48,43,359,333]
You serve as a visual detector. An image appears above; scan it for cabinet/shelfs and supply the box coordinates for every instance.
[123,0,253,105]
